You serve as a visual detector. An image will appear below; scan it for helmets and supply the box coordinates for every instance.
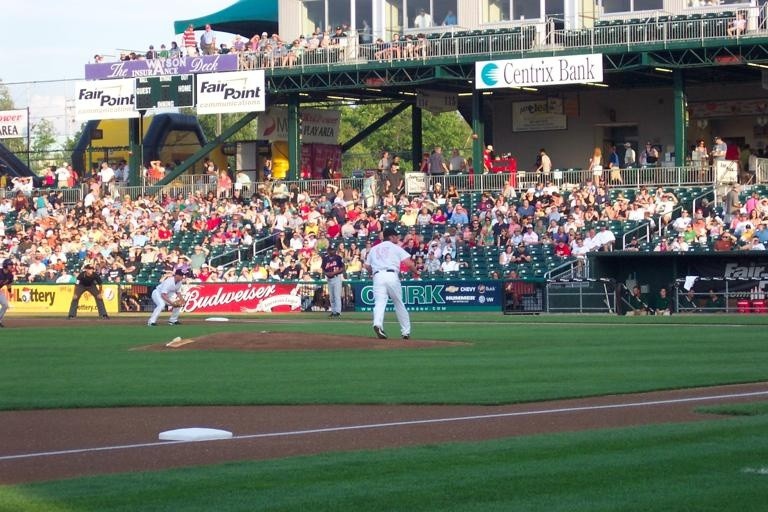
[3,259,14,265]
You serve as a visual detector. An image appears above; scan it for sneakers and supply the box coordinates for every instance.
[401,332,410,339]
[329,311,342,319]
[147,320,183,326]
[373,325,389,339]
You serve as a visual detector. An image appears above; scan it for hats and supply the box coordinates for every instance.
[175,269,187,276]
[383,228,401,237]
[84,264,94,270]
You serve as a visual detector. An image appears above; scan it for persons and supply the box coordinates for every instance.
[727,14,746,38]
[219,19,372,69]
[374,34,431,63]
[629,286,672,315]
[364,228,419,339]
[125,24,217,60]
[147,268,187,326]
[414,8,431,28]
[1,134,768,312]
[442,9,457,27]
[1,260,14,327]
[94,55,103,64]
[321,245,345,318]
[65,264,110,321]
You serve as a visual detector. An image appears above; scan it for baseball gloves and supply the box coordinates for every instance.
[172,300,185,307]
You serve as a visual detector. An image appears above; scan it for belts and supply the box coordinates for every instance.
[373,268,395,275]
